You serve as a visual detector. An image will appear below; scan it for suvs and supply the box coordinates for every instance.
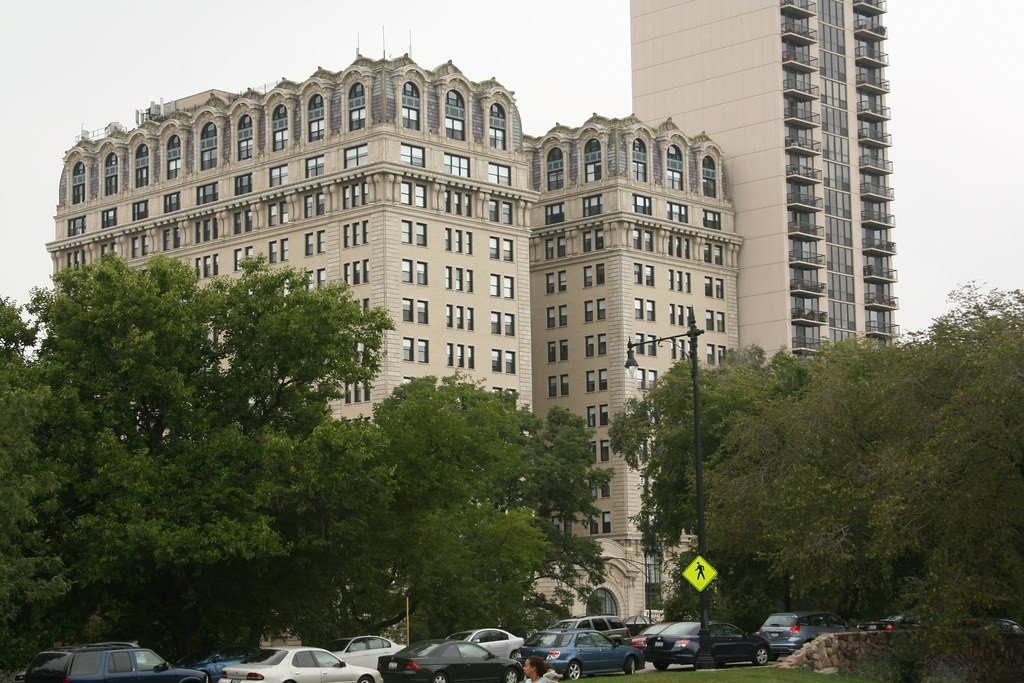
[541,614,632,645]
[12,641,208,683]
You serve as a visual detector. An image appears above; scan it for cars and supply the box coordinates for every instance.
[857,614,1024,635]
[317,636,407,670]
[632,620,771,670]
[218,646,384,683]
[170,645,261,683]
[446,628,525,660]
[516,628,646,680]
[756,611,861,661]
[377,637,524,683]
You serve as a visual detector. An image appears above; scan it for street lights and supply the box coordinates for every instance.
[624,306,717,669]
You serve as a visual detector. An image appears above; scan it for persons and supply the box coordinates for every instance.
[523,656,551,683]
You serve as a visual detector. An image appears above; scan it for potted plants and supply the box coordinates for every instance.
[793,307,827,323]
